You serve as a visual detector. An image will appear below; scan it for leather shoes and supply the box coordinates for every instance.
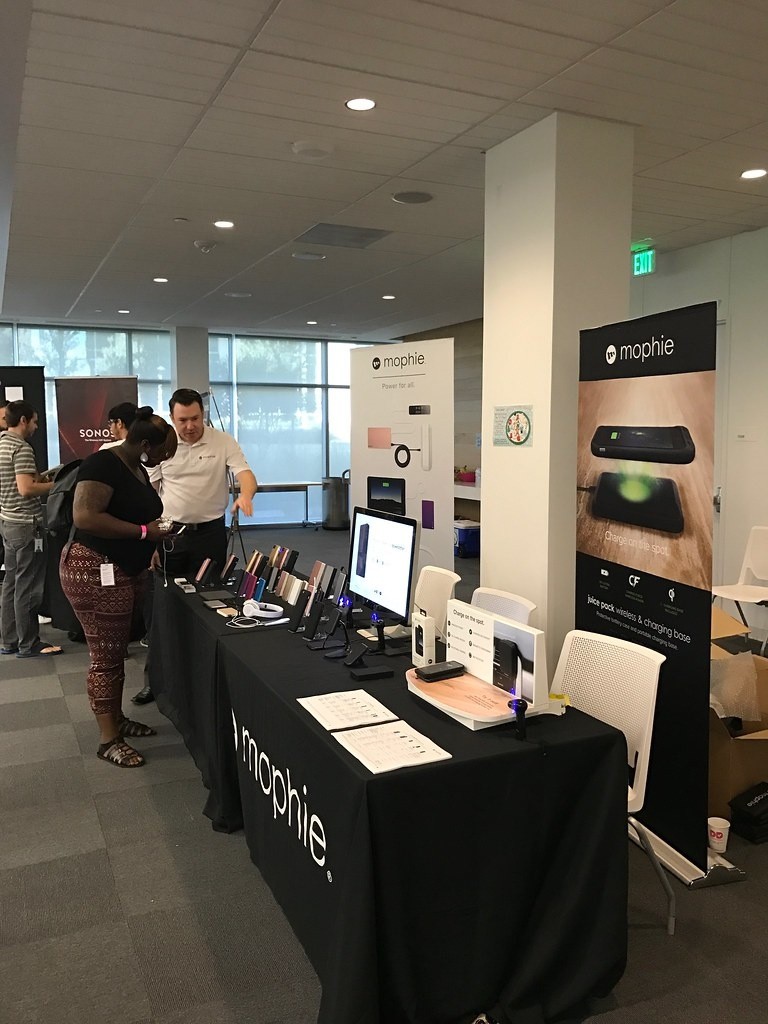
[129,685,156,705]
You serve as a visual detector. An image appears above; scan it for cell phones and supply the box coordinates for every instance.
[422,500,434,529]
[168,524,186,535]
[325,609,342,636]
[591,426,695,465]
[344,644,368,666]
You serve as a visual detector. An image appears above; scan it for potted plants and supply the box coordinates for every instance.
[454,465,475,482]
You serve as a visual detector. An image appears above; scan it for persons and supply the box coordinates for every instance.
[0,399,63,658]
[100,401,155,647]
[130,388,258,706]
[58,405,178,768]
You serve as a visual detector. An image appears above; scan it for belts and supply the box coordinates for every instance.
[175,520,229,533]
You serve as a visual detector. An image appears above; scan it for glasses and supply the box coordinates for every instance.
[107,417,115,422]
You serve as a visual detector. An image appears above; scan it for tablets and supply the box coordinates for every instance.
[367,476,406,516]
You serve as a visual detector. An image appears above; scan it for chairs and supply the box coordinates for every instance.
[549,629,677,935]
[469,586,537,626]
[711,526,768,657]
[413,566,461,642]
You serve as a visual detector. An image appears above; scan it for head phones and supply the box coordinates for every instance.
[243,599,283,618]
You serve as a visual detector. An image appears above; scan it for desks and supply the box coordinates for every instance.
[229,483,323,531]
[148,569,408,834]
[454,481,480,502]
[215,623,628,1024]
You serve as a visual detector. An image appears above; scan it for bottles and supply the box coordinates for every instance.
[475,469,481,487]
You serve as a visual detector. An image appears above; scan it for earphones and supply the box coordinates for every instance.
[164,579,167,587]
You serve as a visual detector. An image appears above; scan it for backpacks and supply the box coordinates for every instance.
[44,458,84,533]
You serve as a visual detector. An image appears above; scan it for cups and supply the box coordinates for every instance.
[709,817,730,853]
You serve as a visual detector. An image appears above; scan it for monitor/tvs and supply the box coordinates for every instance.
[345,506,421,655]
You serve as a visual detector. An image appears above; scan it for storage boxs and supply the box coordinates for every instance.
[727,781,768,845]
[454,520,480,559]
[412,609,435,668]
[708,651,768,822]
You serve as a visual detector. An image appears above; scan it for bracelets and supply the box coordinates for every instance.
[139,525,147,540]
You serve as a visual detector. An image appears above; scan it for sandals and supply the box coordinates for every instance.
[120,718,158,737]
[97,737,146,768]
[0,638,17,653]
[16,641,63,658]
